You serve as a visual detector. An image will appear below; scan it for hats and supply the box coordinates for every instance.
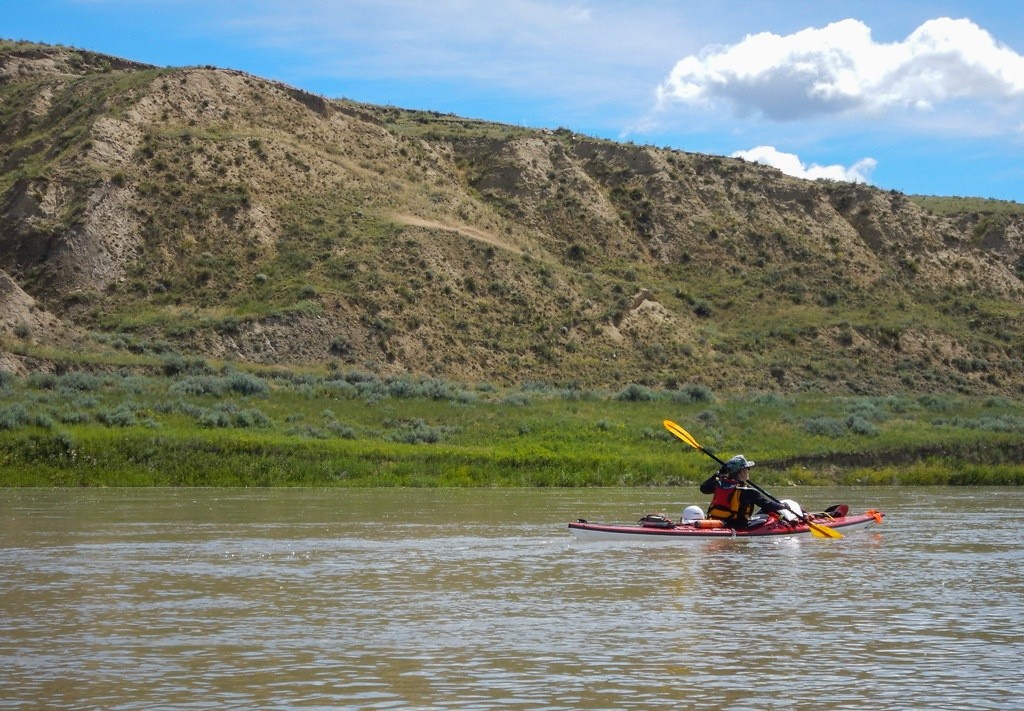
[727,455,755,474]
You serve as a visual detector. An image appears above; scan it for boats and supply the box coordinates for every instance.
[565,503,887,536]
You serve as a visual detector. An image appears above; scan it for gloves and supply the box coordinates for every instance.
[780,502,790,509]
[719,463,729,474]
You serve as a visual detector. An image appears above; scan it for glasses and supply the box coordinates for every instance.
[742,467,750,472]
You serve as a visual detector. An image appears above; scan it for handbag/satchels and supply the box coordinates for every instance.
[638,513,676,529]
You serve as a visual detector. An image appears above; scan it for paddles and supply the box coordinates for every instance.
[663,420,845,540]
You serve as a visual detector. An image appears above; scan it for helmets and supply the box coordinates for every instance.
[682,505,704,524]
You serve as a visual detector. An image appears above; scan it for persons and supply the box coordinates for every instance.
[700,455,789,530]
